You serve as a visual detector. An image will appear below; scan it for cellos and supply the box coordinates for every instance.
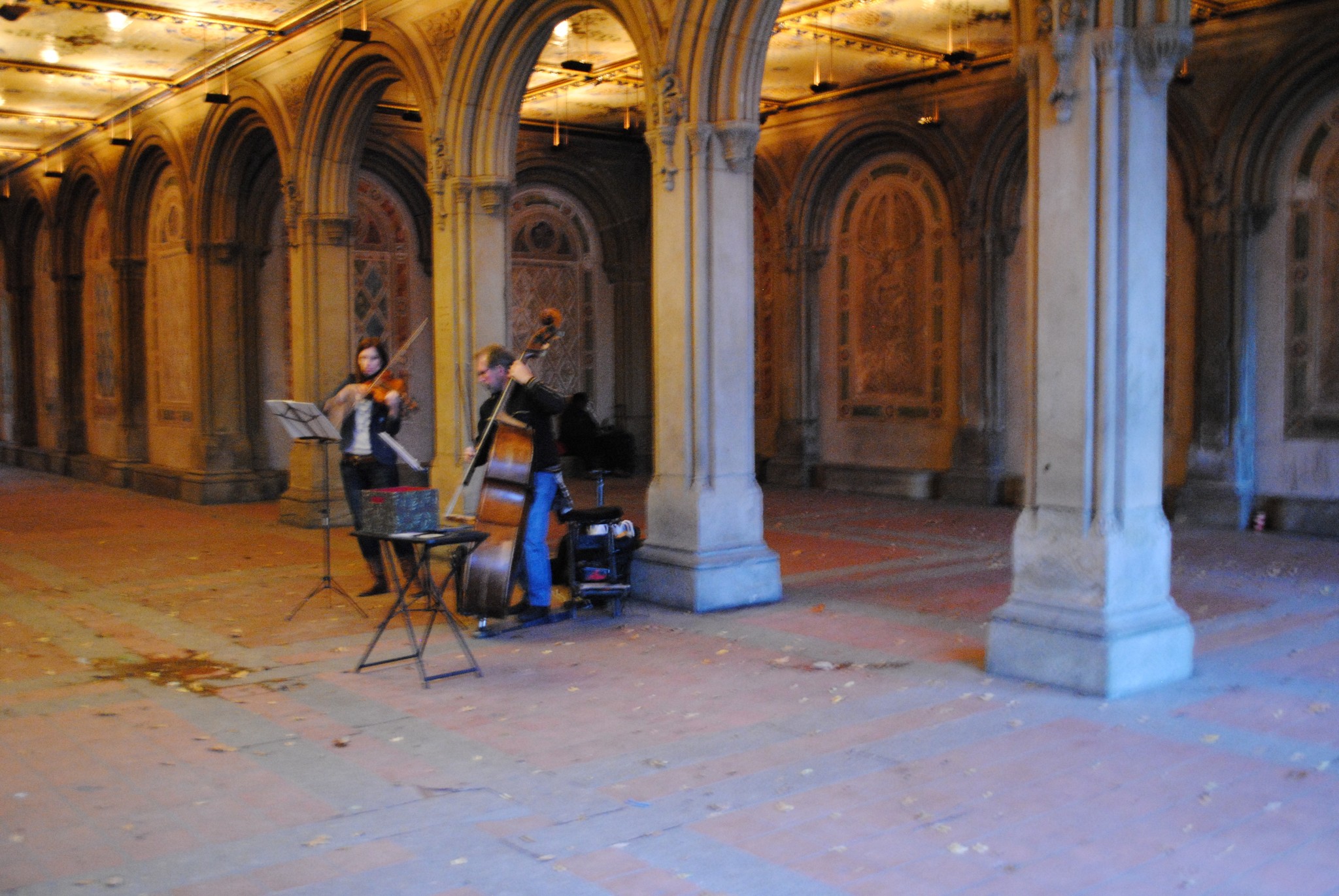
[458,308,566,631]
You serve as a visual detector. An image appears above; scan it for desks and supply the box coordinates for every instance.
[348,527,491,689]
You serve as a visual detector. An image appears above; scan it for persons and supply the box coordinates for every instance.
[461,342,567,624]
[318,337,425,598]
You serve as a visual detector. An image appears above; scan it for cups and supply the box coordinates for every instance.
[590,524,609,535]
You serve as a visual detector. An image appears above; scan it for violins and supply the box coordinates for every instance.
[369,370,420,412]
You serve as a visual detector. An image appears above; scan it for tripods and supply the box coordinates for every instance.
[263,398,372,623]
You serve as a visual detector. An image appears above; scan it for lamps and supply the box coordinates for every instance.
[203,26,231,103]
[43,121,65,178]
[110,80,135,147]
[561,9,592,72]
[808,9,839,94]
[548,87,572,155]
[624,66,644,136]
[942,0,976,66]
[337,0,371,42]
[917,58,944,129]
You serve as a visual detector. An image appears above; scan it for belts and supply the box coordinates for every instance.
[342,454,376,466]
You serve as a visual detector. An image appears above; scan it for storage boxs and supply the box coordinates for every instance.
[361,487,439,535]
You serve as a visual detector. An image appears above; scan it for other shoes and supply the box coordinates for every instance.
[518,605,551,623]
[358,584,389,597]
[407,576,425,598]
[508,600,530,616]
